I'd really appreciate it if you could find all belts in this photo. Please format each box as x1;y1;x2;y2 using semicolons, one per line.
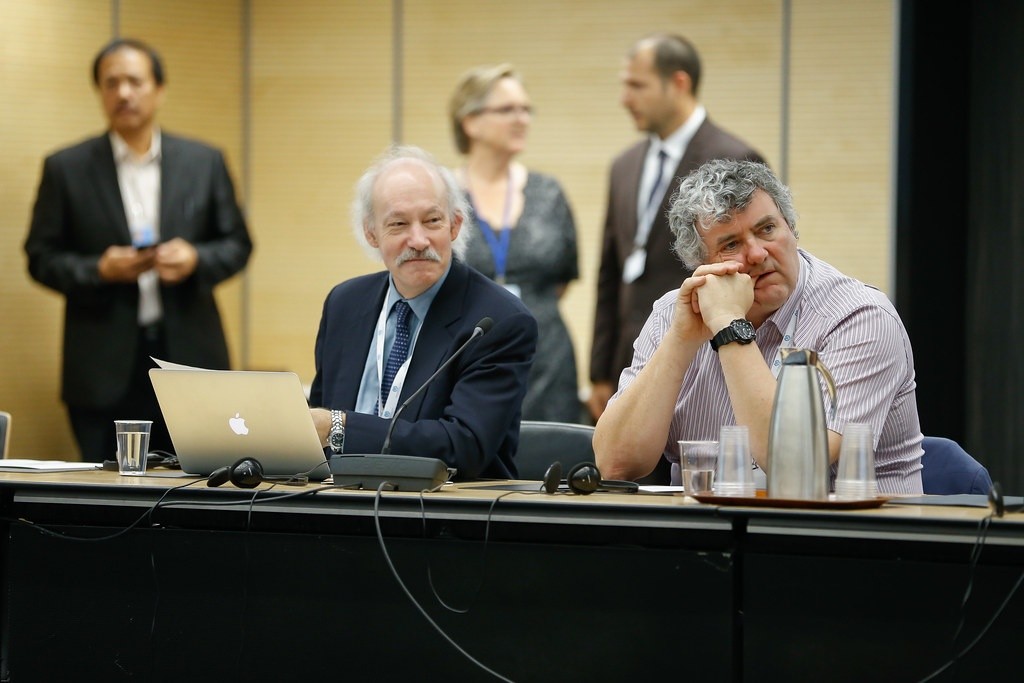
137;325;165;342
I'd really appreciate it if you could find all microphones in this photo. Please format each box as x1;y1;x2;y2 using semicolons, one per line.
330;318;494;491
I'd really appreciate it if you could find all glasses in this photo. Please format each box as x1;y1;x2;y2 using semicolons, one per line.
469;105;532;115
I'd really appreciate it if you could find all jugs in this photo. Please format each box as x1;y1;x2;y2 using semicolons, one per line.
766;348;836;502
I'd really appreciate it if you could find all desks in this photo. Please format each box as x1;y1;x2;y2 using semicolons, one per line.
0;462;1024;683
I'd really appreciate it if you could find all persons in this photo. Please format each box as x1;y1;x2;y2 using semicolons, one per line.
446;65;583;424
308;148;537;482
23;38;253;464
593;160;925;494
588;35;768;428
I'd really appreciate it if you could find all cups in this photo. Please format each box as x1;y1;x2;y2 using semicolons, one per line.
113;420;153;476
834;423;877;501
677;440;718;495
713;426;757;498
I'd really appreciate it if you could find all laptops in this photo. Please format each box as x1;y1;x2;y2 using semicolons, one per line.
148;368;332;482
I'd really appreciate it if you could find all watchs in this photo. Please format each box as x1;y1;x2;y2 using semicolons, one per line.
710;318;758;352
327;410;344;453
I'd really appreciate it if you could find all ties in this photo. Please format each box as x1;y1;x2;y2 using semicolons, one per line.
644;151;666;210
374;301;412;416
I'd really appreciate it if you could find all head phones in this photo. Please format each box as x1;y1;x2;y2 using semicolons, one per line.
206;457;309;489
103;451;183;471
544;462;639;494
986;482;1024;518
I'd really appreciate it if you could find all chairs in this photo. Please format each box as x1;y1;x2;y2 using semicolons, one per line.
919;434;994;496
510;417;668;478
